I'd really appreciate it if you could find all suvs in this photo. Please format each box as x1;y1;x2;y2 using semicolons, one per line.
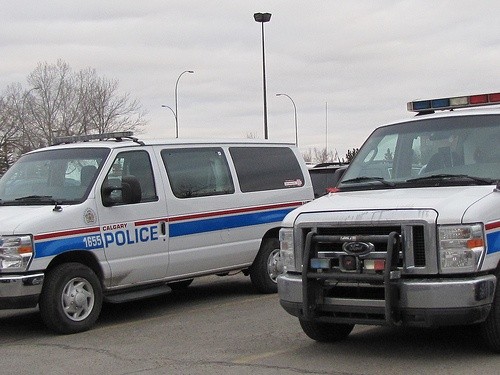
308;161;425;198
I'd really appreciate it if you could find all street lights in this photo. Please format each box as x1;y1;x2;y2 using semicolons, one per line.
253;12;272;138
276;93;298;148
162;70;195;138
21;85;40;154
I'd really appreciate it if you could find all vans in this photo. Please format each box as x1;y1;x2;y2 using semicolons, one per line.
277;93;500;354
0;130;315;334
305;162;319;169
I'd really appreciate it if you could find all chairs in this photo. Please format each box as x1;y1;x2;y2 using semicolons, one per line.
474;132;500;163
70;166;97;198
423;147;463;173
